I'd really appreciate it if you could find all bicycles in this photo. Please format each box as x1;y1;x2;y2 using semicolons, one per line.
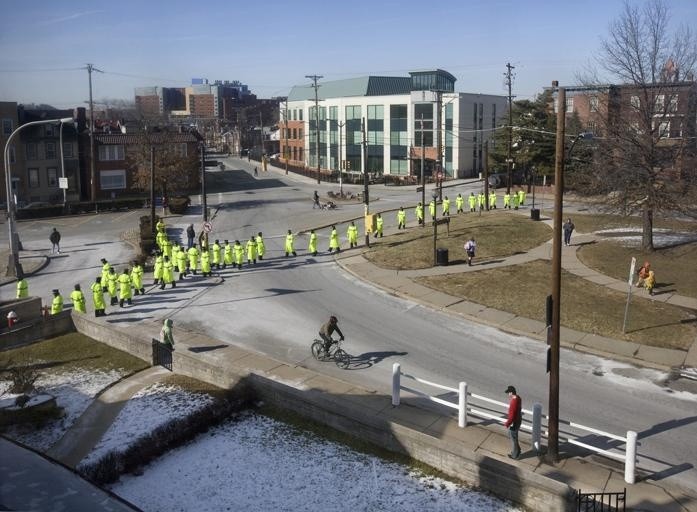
310;336;351;373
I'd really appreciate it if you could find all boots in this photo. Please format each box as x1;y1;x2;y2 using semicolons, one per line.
94;288;145;317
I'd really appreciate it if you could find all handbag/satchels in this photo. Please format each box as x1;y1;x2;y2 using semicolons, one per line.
467;246;475;257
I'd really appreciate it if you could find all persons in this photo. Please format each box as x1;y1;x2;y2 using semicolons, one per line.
643;270;655;295
254;167;258;176
159;318;174;346
51;289;64;314
50;228;61;254
504;386;522;459
563;218;575;247
15;274;29;298
319;316;344;357
635;261;651;288
70;284;86;314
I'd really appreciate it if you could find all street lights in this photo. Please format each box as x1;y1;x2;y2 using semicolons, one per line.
3;116;76;281
143;124;161;232
195;136;209;225
545;82;597;464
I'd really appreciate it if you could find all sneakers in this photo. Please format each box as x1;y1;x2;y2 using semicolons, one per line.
637;267;644;275
564;243;570;246
508;449;521;460
153;255;264;289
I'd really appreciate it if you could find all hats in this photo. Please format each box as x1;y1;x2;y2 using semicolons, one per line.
51;289;59;294
505;386;516;394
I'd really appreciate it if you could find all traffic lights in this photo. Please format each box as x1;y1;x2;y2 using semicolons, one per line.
432;171;436;181
347;160;350;170
438;172;441;182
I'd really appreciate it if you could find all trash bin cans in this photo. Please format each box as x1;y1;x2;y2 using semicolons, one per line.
531;209;539;220
437;248;448;266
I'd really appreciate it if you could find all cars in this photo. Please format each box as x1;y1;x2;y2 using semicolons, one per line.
270;153;279;160
